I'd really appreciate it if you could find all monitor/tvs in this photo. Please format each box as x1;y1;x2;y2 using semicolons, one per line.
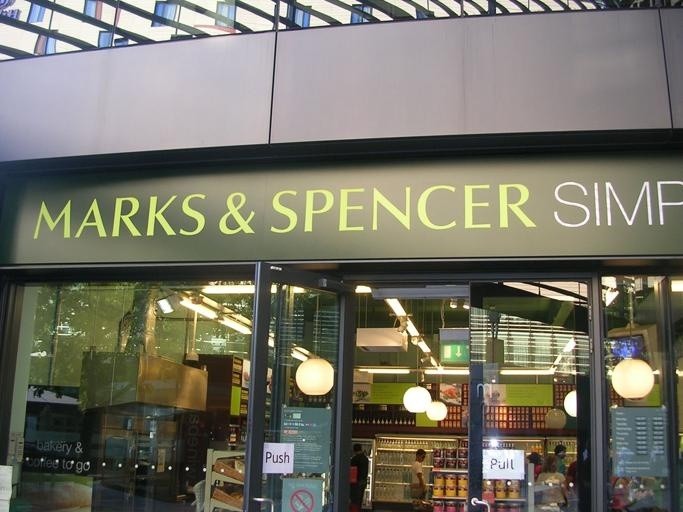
605;329;657;376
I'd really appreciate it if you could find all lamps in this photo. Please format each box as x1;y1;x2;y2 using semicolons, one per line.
176;289;313;363
396;312;431;364
424;374;447;422
384;297;431;354
610;276;655;401
563;388;577;418
294;291;334;397
402;299;432;415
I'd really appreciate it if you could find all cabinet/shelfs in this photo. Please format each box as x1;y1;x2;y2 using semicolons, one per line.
430;463;534;512
204;447;245;512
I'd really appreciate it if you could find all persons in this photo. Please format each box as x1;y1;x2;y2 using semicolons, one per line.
184;472;205;508
411;449;427;499
350;443;368;508
527;444;579;511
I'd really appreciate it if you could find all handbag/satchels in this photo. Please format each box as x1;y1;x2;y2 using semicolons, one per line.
350;466;358;484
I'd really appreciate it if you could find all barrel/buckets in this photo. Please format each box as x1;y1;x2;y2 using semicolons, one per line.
432;445;521;512
